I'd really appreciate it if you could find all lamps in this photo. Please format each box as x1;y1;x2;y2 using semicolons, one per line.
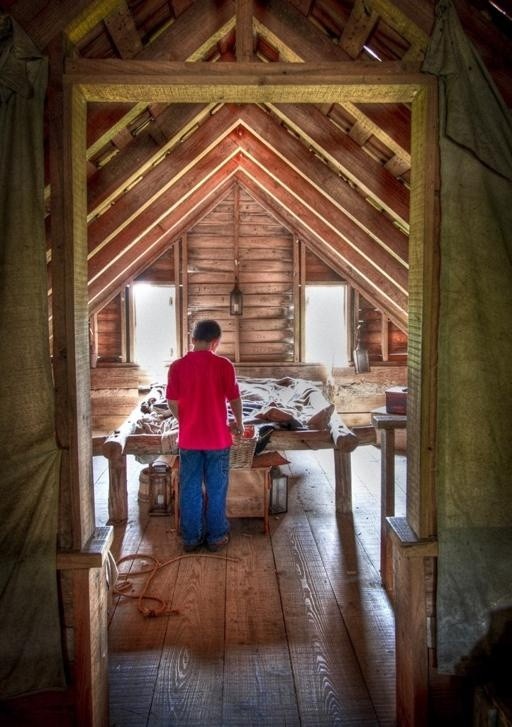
229;278;244;315
351;318;370;374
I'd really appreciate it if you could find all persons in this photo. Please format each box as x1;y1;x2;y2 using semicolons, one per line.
167;319;244;552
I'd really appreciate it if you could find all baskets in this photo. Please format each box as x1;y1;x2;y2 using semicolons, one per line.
230;425;259;469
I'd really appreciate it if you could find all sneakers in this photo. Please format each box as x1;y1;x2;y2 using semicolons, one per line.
185;534;230;552
